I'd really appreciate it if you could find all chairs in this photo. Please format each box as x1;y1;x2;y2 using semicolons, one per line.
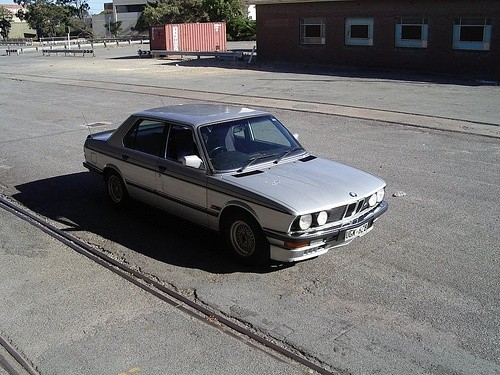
206;126;236;157
170;128;194;161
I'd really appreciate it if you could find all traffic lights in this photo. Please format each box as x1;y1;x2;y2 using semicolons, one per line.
65;26;70;33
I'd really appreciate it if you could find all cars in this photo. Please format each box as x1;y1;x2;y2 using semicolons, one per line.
82;104;389;265
